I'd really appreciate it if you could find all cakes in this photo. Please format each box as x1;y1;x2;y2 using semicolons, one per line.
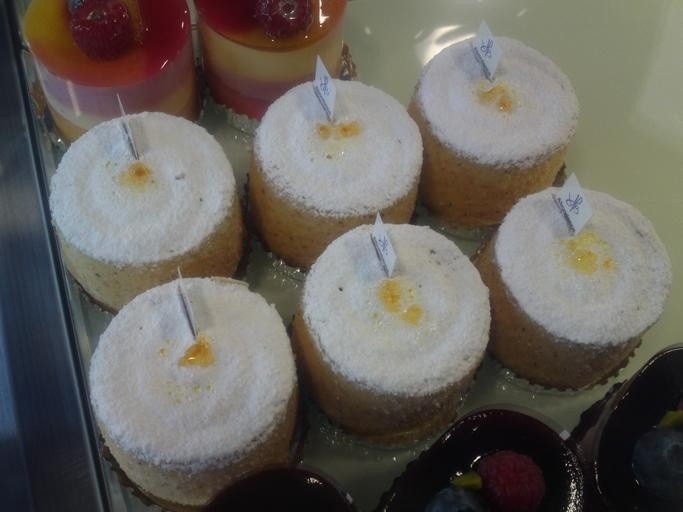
289;224;490;444
86;277;298;507
193;0;350;137
20;0;202;148
469;186;673;393
404;37;581;230
198;461;353;512
47;111;250;322
570;343;683;512
374;406;587;512
251;79;425;275
